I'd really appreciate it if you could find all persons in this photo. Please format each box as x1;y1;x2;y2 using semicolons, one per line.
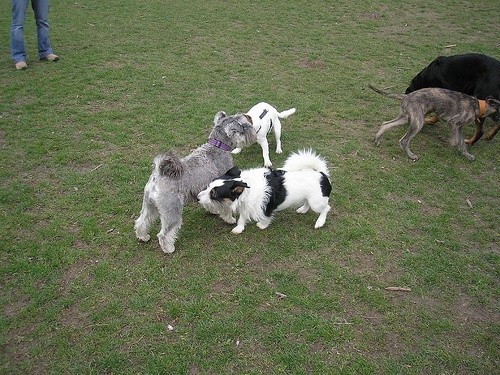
10;0;59;70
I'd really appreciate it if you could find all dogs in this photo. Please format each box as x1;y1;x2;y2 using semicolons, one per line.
366;84;500;162
196;146;332;235
133;111;258;254
231;101;297;168
405;53;500;147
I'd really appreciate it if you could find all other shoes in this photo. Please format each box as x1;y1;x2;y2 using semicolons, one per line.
15;61;27;70
46;53;60;62
